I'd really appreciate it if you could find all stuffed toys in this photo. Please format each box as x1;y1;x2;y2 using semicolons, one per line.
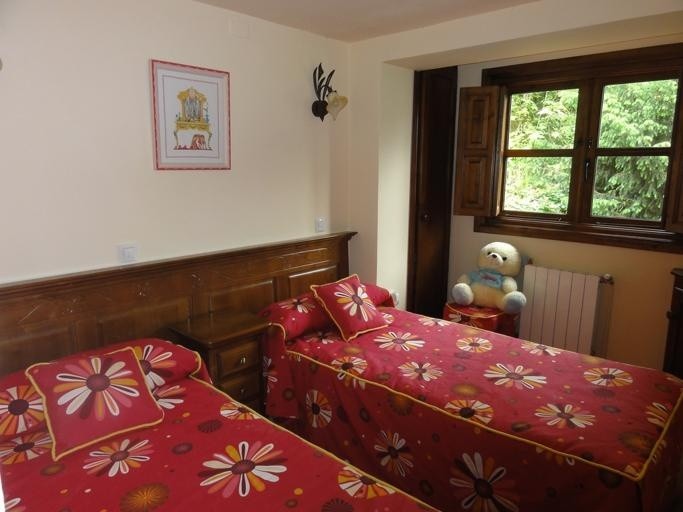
451;240;527;314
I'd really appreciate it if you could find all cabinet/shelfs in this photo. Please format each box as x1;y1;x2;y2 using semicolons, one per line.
161;308;273;411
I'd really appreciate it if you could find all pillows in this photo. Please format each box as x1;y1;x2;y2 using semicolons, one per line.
259;282;389;342
1;337;199;439
26;346;166;460
310;273;387;338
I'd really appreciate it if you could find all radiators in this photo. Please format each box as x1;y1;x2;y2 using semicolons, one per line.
518;256;615;356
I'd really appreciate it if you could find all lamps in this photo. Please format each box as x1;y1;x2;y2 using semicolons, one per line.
310;61;348;122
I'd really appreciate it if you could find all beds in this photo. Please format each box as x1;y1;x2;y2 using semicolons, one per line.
261;308;683;511
2;376;437;511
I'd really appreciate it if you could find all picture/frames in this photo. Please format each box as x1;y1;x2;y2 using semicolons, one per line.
150;58;231;171
